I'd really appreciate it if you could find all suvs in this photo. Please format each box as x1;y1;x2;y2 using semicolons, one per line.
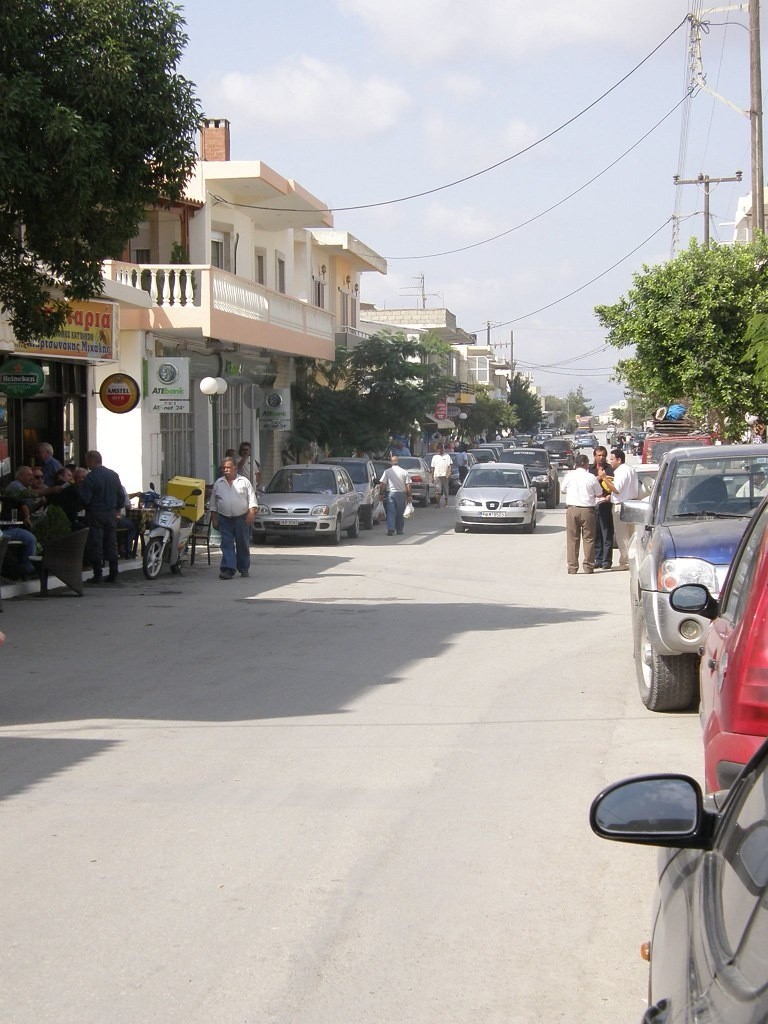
492;447;560;508
540;438;581;470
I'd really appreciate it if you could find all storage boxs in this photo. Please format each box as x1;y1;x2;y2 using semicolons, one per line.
167;475;206;521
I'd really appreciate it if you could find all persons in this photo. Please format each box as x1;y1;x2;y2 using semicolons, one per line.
0;441;136;586
236;442;262;491
431;436;468;452
645;429;656;438
617;432;634;454
754;424;768;462
735;464;768;498
560;445;639;574
209;457;258;580
215;449;236;477
395;442;411;457
454;448;468;483
430;443;453;508
354;448;369;460
379;456;413;536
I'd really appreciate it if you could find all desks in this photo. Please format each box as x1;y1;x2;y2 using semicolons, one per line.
127;508;157;559
0;521;23;568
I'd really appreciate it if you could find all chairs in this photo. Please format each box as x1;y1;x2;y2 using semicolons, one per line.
188;507;212;566
306;473;331;490
678;476;728;514
351;471;364;481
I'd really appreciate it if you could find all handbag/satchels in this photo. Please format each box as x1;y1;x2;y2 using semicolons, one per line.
403;501;414;519
373;501;387;521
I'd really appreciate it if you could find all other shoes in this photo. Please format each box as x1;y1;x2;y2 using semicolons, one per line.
110;576;122;582
387;530;393;536
241;571;249;578
396;531;405;535
612;565;630;571
86;575;104;584
602;564;609;569
220;569;234;579
593;564;601;569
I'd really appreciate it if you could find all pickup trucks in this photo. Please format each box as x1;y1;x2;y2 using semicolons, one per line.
620;440;768;713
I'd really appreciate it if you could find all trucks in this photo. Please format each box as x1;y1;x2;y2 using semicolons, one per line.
576;415;594;434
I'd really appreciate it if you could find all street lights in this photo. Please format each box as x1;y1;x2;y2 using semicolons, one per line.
458;412;468;441
200;376;228;546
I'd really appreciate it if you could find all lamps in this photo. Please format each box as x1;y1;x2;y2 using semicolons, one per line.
319;265;326;280
352;283;359;297
343;275;350;289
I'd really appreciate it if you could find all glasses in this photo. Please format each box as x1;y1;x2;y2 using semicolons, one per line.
33;475;46;480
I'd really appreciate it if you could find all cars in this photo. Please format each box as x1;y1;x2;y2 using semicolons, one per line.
251;463;363;545
395;456;436;507
589;732;767;1024
606;419;717;536
577;434;600;449
667;493;768;795
424;426;571;493
453;461;540;534
371;459;392;505
313;457;382;529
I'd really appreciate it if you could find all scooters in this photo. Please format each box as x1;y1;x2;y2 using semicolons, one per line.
136;482;202;580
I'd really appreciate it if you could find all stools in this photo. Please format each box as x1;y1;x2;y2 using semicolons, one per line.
29;556;48;597
115;528;130;560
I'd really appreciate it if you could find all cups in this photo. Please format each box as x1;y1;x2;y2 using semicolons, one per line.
11;509;18;523
